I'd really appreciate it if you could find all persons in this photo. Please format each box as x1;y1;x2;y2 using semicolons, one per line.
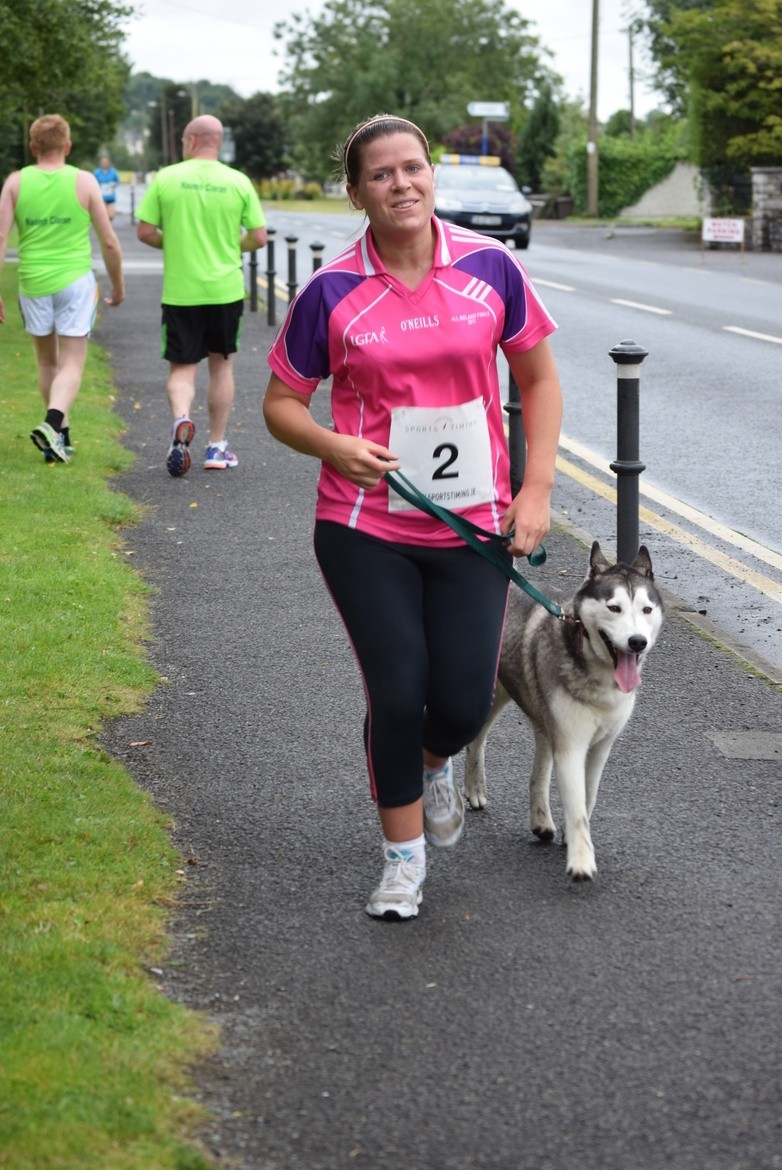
87;154;121;222
1;112;127;470
135;112;268;477
256;114;566;926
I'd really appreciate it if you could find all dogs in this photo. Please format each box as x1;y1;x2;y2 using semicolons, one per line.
460;537;663;882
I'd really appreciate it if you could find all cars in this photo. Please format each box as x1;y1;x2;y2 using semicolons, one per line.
431;154;534;249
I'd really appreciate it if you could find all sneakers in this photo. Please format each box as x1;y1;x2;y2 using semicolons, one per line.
422;755;465;849
364;853;427;922
166;413;196;478
204;444;239;469
30;420;75;465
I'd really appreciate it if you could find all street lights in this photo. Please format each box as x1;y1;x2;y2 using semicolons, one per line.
147;85;198;167
